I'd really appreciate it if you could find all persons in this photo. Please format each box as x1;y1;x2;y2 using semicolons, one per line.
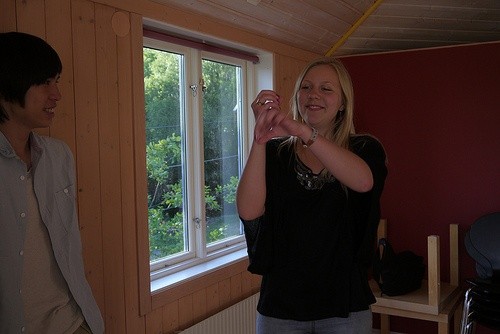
0;31;106;334
237;59;388;334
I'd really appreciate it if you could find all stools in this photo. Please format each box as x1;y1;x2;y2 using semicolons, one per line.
371;290;464;334
369;219;463;316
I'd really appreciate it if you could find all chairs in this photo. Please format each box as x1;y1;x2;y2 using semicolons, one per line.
460;212;500;334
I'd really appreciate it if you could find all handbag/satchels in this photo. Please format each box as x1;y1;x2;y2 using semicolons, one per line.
372;238;424;297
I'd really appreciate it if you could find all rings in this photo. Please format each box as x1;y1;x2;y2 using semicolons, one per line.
256;102;264;106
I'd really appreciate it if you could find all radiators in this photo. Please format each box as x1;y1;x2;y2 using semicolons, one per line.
177;292;261;334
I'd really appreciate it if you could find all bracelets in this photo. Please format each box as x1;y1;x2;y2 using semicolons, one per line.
301;127;319;151
